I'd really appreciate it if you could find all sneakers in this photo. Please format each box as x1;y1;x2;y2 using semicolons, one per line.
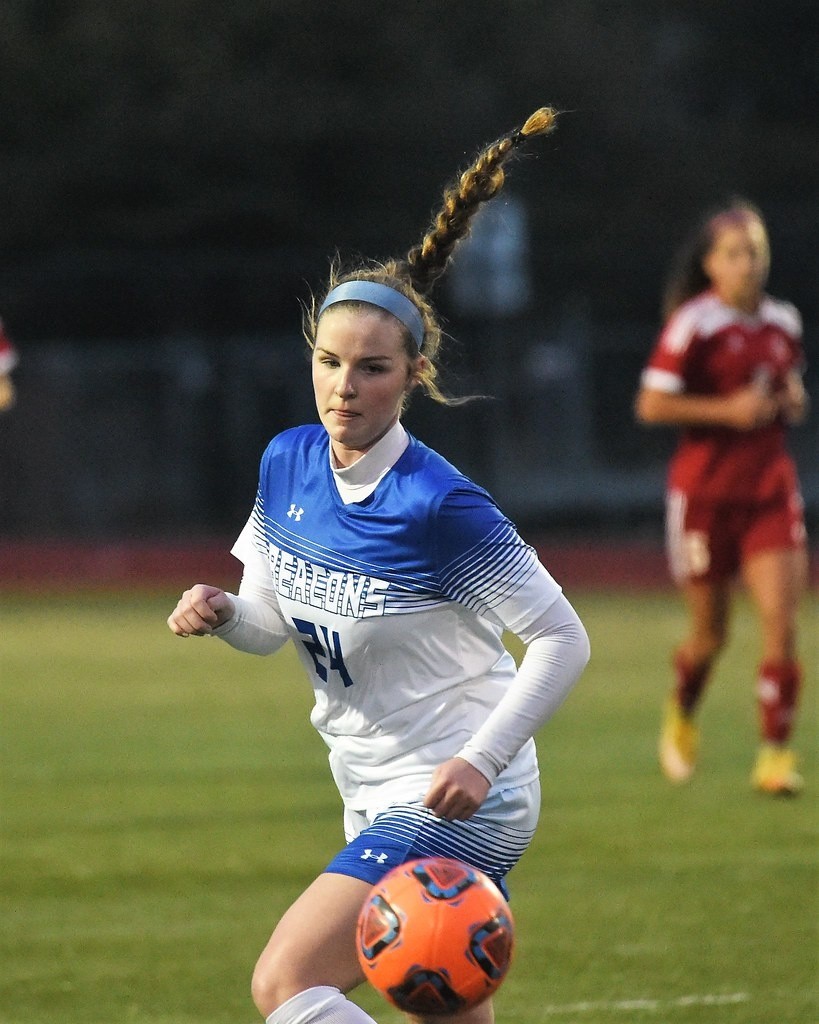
659;699;696;782
753;747;803;795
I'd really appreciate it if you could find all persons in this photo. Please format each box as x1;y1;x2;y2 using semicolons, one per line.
635;200;811;798
166;106;590;1024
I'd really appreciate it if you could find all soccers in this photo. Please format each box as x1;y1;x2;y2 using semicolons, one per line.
357;858;518;1016
